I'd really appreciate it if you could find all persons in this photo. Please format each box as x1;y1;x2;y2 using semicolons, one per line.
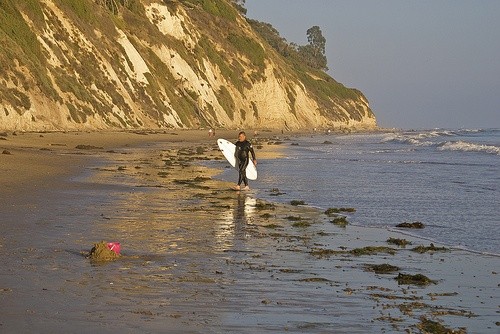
208;128;216;137
234;132;258;191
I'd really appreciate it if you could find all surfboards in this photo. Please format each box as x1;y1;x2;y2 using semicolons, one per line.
217;138;257;181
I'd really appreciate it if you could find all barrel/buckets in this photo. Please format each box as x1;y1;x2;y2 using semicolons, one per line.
107;242;120;255
